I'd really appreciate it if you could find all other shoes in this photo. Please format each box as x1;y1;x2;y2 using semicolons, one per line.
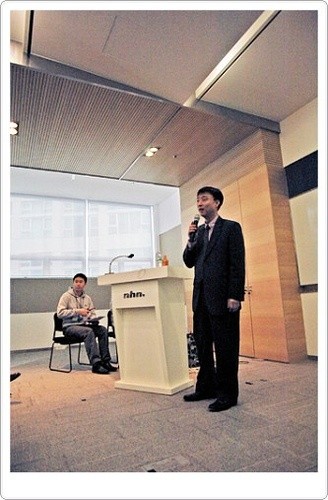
102;362;119;370
92;365;110;374
208;400;235;412
10;372;22;381
183;393;213;402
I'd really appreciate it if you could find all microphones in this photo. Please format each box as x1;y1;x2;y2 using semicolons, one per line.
189;215;199;242
109;254;134;273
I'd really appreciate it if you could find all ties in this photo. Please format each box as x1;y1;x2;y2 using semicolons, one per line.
202;224;211;255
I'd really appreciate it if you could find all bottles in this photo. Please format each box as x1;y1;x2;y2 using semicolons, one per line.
156;251;162;267
162;255;169;266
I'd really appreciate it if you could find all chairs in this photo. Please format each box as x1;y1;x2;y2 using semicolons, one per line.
49;309;118;373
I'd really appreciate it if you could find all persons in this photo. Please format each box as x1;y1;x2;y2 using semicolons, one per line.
57;273;117;374
183;186;245;412
